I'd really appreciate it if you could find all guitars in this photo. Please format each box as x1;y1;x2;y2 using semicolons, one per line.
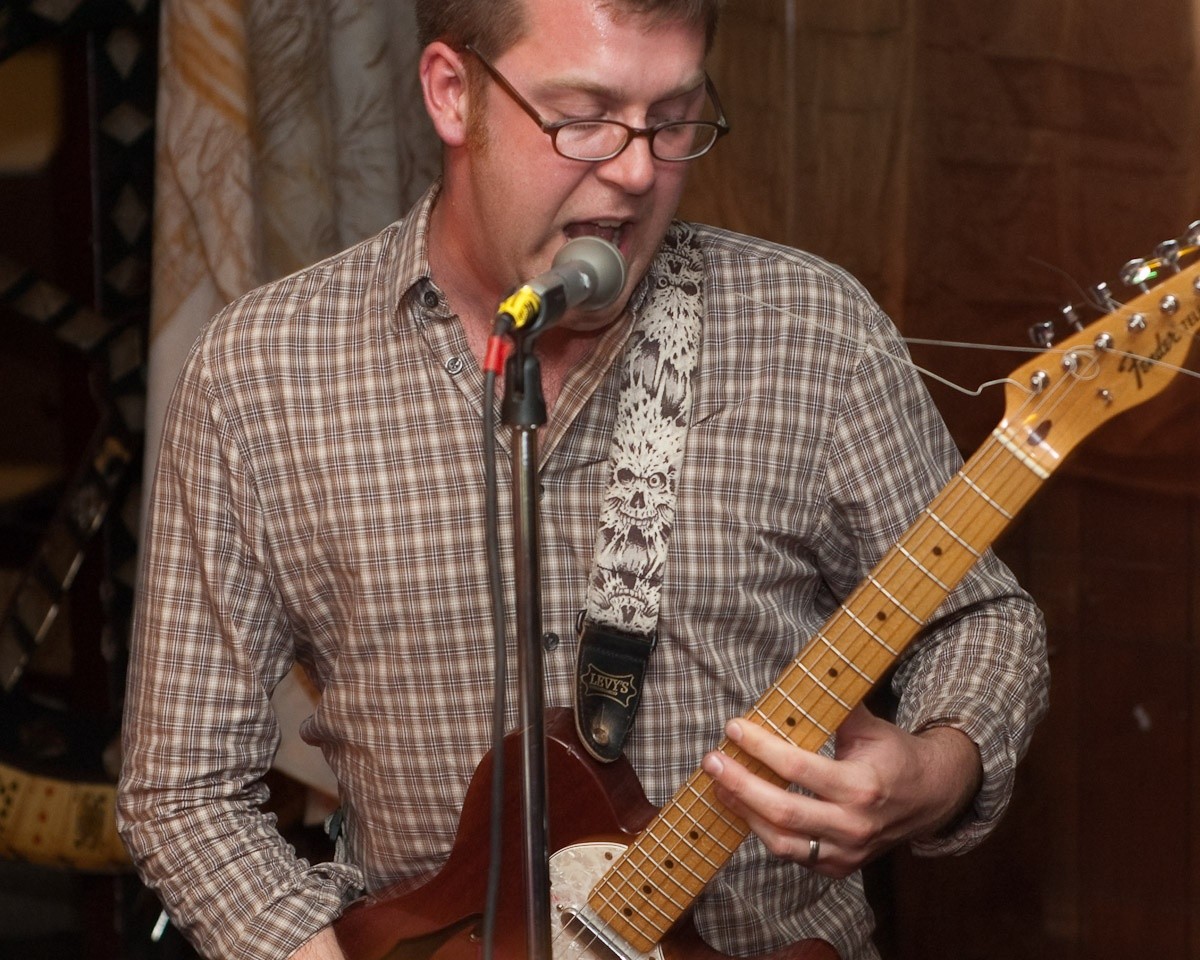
331;219;1200;960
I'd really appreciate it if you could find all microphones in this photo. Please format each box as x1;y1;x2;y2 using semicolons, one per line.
498;236;628;332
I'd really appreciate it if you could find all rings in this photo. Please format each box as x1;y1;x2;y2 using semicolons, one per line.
805;838;819;867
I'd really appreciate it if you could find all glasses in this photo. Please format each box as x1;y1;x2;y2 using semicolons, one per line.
448;42;729;164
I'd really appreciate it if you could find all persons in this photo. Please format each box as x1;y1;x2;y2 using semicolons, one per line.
111;0;1048;960
